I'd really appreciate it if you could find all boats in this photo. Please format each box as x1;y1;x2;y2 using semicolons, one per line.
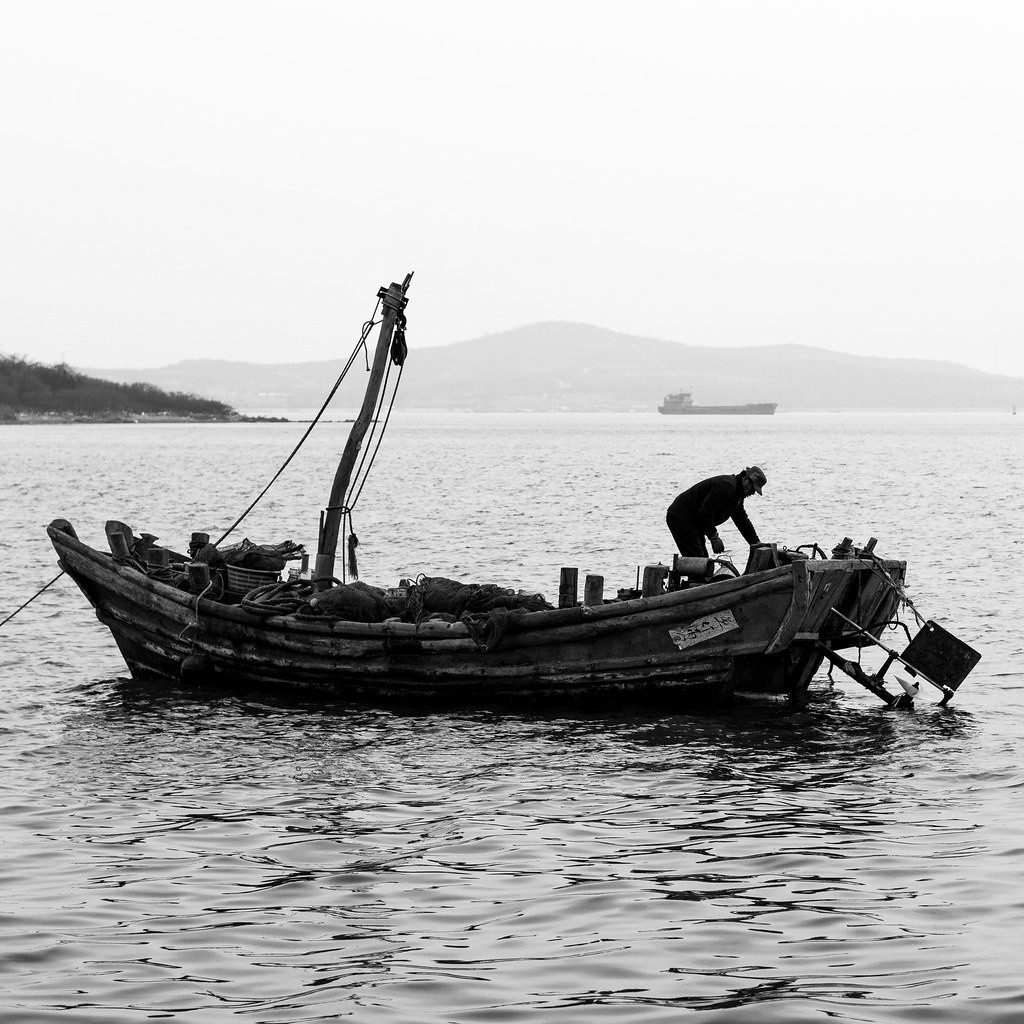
657;391;780;416
44;271;984;721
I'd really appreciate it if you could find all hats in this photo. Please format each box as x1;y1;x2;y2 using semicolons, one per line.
744;466;767;496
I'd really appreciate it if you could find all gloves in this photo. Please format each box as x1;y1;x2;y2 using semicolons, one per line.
710;532;725;554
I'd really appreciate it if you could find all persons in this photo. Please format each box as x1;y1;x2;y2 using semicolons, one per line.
666;466;768;581
